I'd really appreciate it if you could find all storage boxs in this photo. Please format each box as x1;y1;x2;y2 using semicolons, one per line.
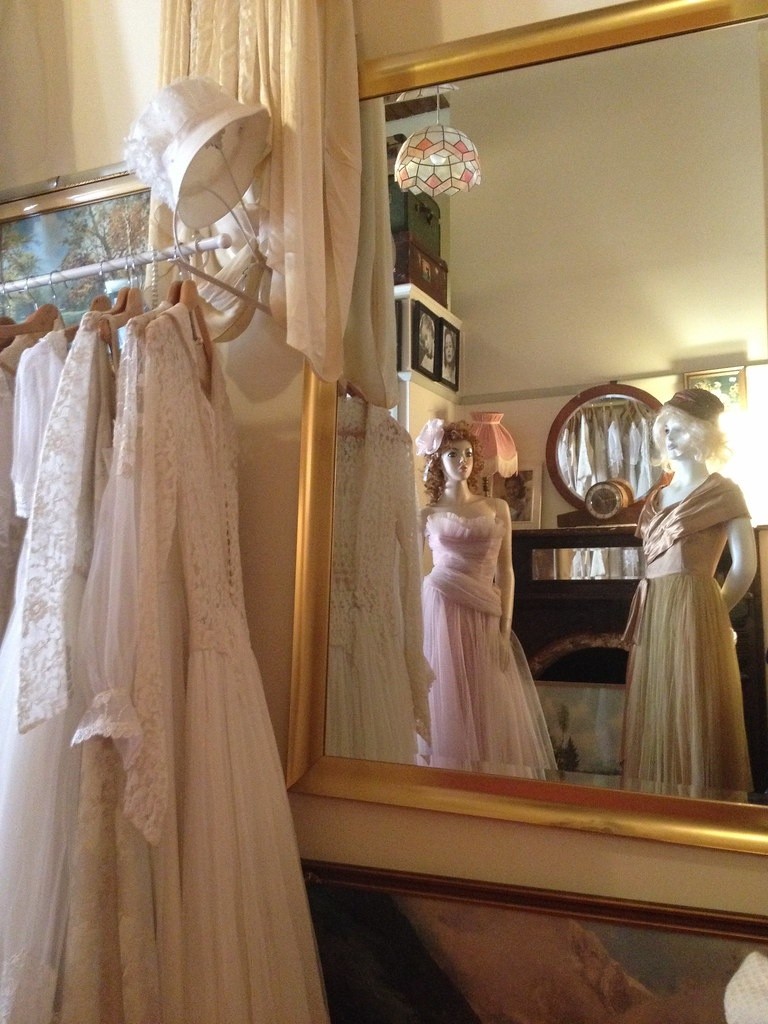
387;229;450;311
388;173;443;261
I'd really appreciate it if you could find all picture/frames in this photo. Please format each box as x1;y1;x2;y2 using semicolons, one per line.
490;466;545;531
0;165;166;348
684;364;748;410
438;316;462;388
412;298;442;378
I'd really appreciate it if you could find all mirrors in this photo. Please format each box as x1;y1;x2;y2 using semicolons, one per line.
289;0;764;841
548;378;676;530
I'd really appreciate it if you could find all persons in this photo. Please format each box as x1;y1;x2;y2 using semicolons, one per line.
418;417;556;771
622;388;756;794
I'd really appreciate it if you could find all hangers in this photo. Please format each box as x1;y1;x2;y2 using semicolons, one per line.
560;394;658;444
337;369;416;447
2;242;236;413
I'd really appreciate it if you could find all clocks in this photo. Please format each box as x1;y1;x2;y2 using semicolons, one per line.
555;481;642;526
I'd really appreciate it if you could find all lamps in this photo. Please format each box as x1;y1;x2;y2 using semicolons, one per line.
390;80;481;204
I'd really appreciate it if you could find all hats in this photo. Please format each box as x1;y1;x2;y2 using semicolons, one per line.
668;389;724;419
126;77;270;229
